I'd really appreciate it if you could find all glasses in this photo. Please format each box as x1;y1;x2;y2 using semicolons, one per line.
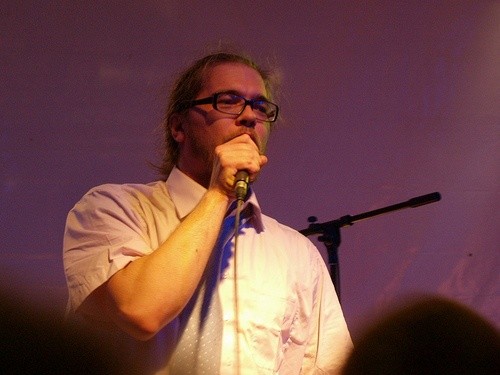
178;93;279;122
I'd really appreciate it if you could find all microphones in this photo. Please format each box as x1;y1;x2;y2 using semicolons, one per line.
235;170;250;200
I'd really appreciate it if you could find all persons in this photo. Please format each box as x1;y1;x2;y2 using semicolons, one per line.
334;294;500;375
63;50;356;375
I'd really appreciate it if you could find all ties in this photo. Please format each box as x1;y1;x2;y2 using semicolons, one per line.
163;215;253;375
